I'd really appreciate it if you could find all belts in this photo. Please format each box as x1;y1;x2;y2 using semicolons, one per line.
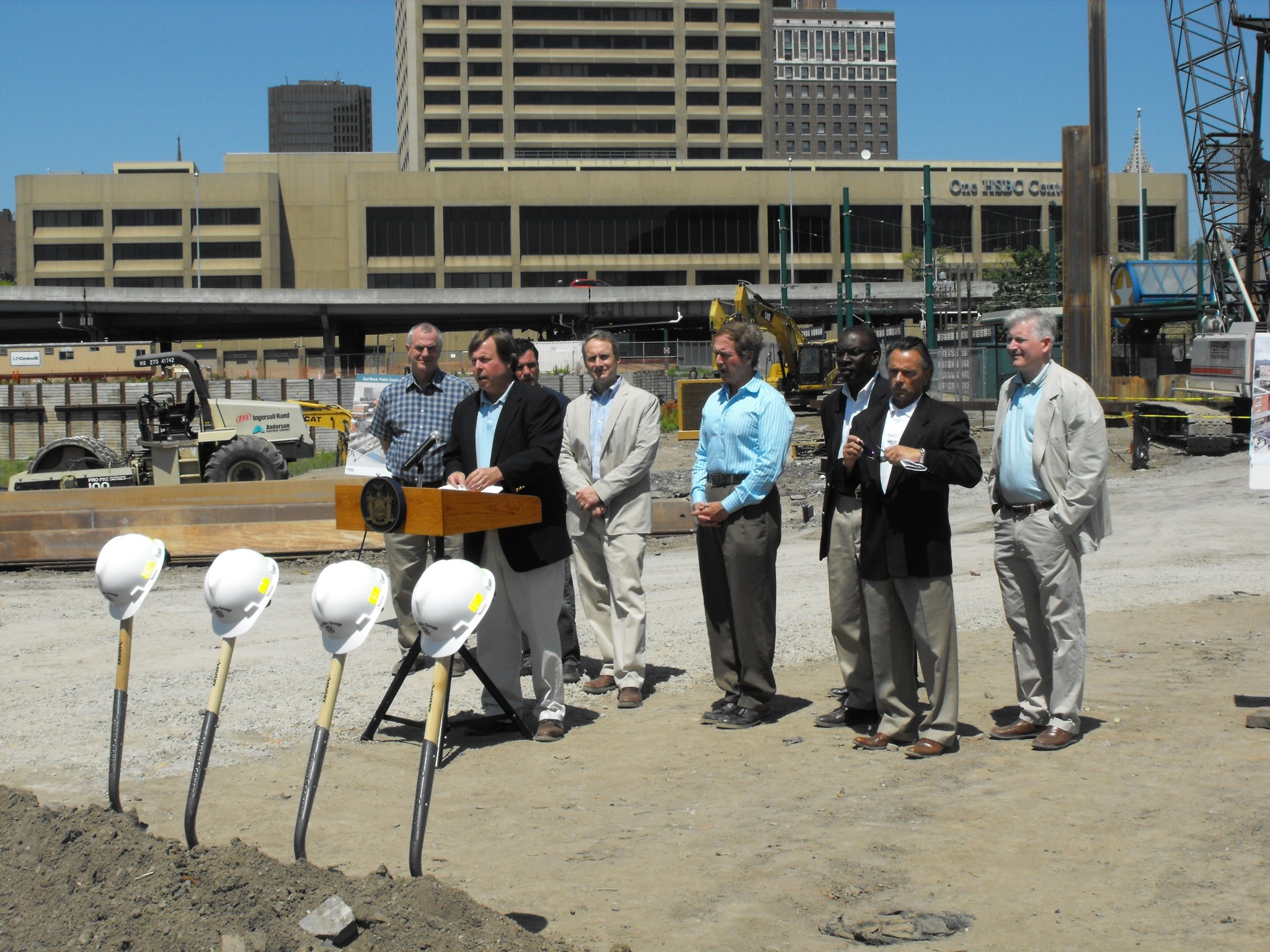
1001;499;1053;513
392;476;447;489
705;473;745;487
838;489;862;500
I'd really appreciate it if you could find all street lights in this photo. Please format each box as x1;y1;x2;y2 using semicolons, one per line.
788;158;794;284
194;169;201;289
1136;108;1143;260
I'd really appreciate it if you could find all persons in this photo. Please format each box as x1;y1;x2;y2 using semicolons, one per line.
514;338;581;684
813;324;919;728
688;322;795;728
367;322;476;676
989;307;1114;750
828;336;983;758
442;327;573;741
557;329;661;708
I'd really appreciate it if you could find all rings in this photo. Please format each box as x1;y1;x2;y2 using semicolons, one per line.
891;455;896;459
479;481;483;485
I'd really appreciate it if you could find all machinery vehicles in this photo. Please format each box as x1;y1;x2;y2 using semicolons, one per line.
6;353;354;491
1131;1;1270;470
676;282;842;439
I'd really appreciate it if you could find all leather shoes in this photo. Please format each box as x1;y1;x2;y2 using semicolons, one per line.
904;738;947;758
464;716;520;736
1031;725;1080;750
699;701;739;724
852;732;913;750
535;719;564;742
827;687;850;697
813;706;870;727
989;718;1047;739
617;687;643;708
583;675;617;693
716;702;773;729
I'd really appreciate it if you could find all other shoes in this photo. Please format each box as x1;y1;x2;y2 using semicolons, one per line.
392;656;427;675
452;658;467;676
520;655;533;676
562;665;581;683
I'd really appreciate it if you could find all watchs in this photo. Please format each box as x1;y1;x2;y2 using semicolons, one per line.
919;448;925;464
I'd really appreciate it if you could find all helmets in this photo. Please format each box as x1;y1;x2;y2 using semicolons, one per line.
95;533;165;621
204;548;280;638
411;559;496;657
310;560;390;653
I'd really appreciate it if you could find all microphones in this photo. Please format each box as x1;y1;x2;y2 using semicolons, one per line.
402;430;443;472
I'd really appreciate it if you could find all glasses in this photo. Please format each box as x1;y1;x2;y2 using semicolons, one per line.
857;420;888;462
830;348;874;359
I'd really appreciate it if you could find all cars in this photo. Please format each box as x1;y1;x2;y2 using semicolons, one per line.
569;278;609;287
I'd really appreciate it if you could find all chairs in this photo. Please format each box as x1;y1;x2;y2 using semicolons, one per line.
159;388;195;429
